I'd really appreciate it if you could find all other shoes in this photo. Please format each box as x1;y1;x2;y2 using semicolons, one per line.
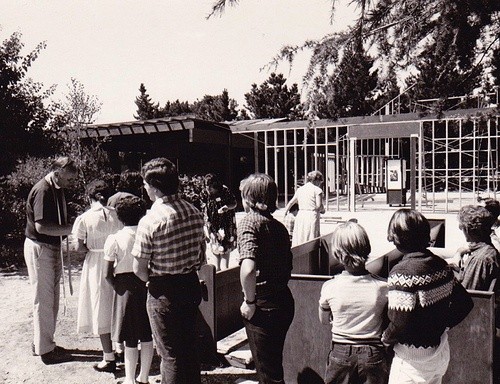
94;359;116;373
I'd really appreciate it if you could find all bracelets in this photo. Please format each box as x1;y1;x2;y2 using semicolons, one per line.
243;296;258;304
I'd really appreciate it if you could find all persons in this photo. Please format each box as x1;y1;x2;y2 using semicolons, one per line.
236;173;295;384
284;203;300;248
23;157;80;364
390;170;398;181
199;173;237;272
317;221;389;384
131;158;207;384
283;171;326;249
106;170;144;202
71;179;125;372
104;195;154;384
479;198;500;252
447;205;500;384
380;208;474;384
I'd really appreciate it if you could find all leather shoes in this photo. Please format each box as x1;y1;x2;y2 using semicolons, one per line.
41;347;74;365
